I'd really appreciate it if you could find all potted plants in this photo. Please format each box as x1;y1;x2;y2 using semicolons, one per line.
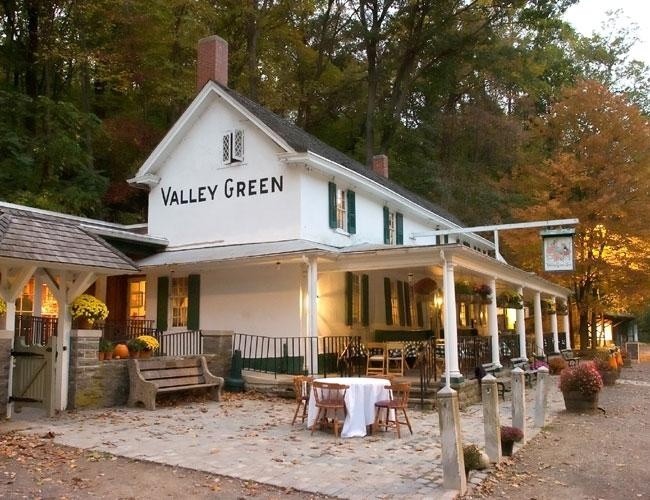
128;335;160;359
500;426;524;456
548;357;568;375
68;294;110;329
463;443;482;482
557;361;604;413
530;360;550;379
590;347;631;385
99;337;113;361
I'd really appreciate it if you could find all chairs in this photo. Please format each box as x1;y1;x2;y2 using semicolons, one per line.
291;338;445;444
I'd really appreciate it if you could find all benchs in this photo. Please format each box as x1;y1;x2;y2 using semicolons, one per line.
560;349;580;370
481;363;511;401
511;357;538;389
127;356;224;411
